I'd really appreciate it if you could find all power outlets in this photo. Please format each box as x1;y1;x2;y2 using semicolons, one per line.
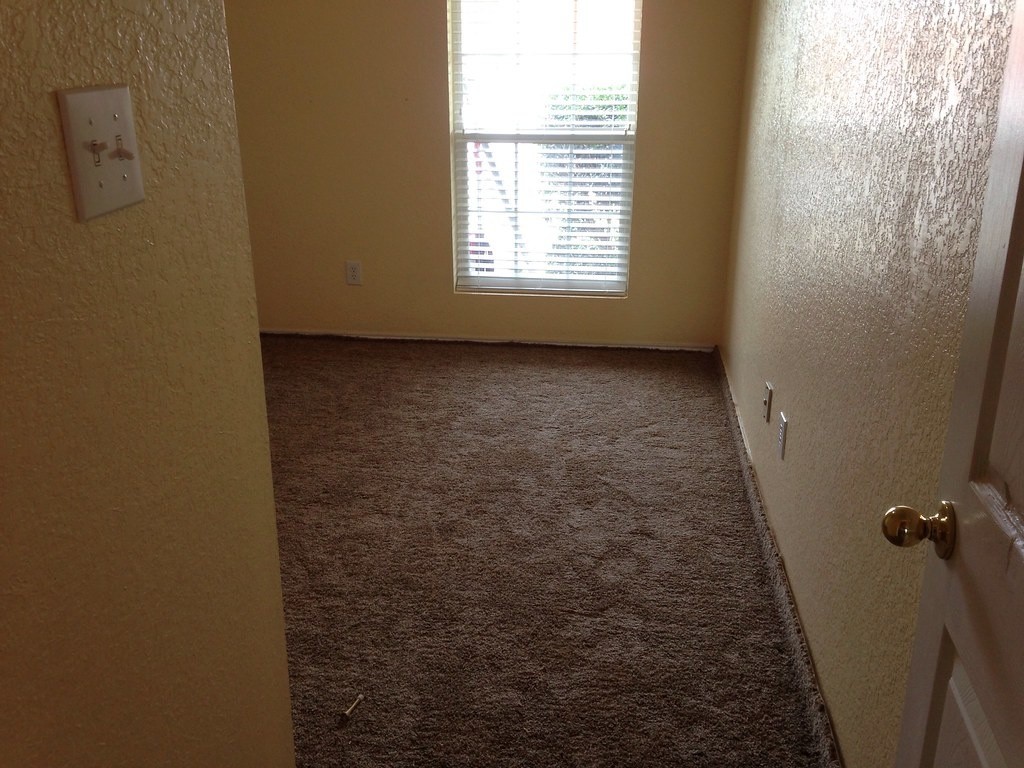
347;260;362;285
777;412;787;459
